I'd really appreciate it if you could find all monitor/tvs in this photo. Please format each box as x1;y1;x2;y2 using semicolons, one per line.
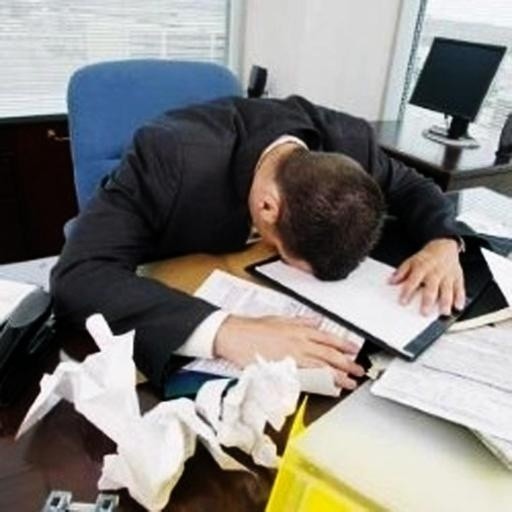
408;36;507;147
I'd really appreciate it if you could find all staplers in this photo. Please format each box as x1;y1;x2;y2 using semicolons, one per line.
0;289;54;389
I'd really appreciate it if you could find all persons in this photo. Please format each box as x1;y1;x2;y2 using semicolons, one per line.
49;95;467;390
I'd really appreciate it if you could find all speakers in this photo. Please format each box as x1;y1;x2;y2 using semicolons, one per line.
247;64;267;97
495;112;512;157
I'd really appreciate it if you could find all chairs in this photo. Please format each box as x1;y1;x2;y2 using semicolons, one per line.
66;58;244;214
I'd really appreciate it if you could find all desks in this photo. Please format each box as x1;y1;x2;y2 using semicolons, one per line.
369;118;512;200
0;237;347;512
